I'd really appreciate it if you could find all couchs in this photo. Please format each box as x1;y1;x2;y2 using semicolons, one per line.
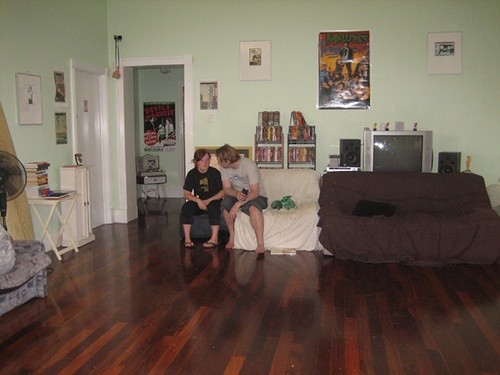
317;171;500;266
0;224;52;317
486;183;500;217
230;169;324;251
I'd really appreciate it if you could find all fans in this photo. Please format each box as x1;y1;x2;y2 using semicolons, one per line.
0;150;28;233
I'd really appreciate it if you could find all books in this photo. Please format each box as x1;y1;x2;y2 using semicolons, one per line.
256;145;284;166
287;145;314;167
44;190;77;199
254;111;285;144
287;110;316;143
23;161;50;200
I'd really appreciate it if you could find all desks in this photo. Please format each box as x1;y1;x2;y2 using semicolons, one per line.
28;192;80;263
136;170;167;203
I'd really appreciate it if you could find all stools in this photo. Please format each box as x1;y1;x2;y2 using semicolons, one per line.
179;210;230;242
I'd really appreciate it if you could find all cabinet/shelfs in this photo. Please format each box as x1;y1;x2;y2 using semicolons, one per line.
286;132;317;171
254;133;284;169
59;166;96;247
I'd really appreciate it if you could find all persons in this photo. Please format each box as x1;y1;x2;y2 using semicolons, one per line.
215;143;268;254
180;149;224;248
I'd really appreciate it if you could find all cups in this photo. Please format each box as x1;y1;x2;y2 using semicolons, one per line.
241;188;248;201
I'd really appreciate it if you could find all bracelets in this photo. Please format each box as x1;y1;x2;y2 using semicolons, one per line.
235;191;239;199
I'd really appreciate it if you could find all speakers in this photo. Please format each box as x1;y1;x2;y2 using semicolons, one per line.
339;138;361;167
438;151;461;173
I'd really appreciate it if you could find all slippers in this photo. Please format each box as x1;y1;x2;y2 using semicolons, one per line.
202;241;219;248
184;240;195;248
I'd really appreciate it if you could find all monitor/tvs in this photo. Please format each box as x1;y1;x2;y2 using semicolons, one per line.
363;131;432;172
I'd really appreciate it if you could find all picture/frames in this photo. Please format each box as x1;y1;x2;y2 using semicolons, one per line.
15;73;44;126
239;40;272;82
52;111;69;147
427;31;463;76
194;146;253;161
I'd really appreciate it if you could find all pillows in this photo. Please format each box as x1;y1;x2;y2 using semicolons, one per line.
351;200;397;218
442;190;483;216
